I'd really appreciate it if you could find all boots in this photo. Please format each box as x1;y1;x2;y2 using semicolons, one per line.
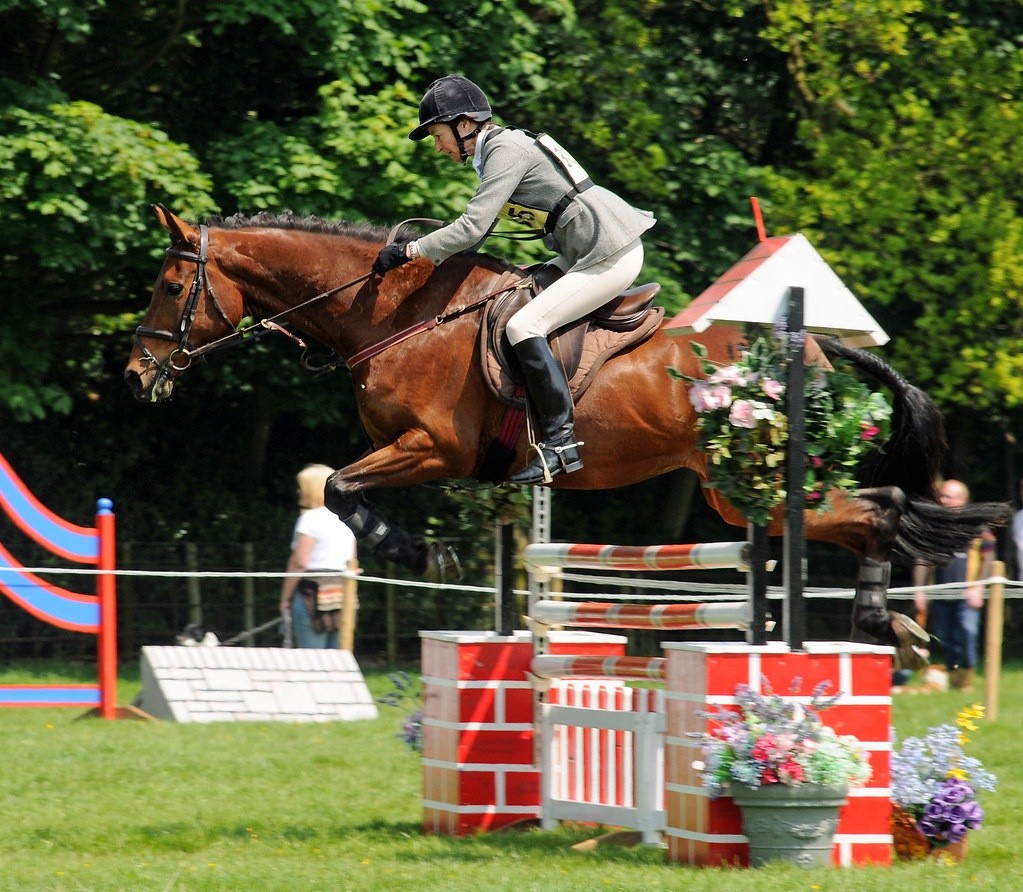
505;336;585;483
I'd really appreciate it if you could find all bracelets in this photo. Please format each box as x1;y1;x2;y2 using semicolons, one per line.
409;241;415;259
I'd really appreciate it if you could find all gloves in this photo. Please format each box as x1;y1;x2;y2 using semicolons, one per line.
372;241;413;277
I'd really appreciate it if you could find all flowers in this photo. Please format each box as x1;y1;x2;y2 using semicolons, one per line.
687;671;872;791
879;710;999;841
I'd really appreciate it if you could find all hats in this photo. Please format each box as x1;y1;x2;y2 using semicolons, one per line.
408;74;492;140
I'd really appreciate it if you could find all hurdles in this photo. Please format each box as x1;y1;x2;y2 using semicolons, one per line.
520;476;771;854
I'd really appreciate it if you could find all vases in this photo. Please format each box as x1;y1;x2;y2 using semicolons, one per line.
730;784;847;865
890;825;969;864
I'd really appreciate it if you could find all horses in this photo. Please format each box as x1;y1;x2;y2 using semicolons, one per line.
121;202;1015;650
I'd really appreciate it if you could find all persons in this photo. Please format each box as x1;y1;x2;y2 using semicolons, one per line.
913;480;1023;694
370;78;657;486
279;464;358;648
